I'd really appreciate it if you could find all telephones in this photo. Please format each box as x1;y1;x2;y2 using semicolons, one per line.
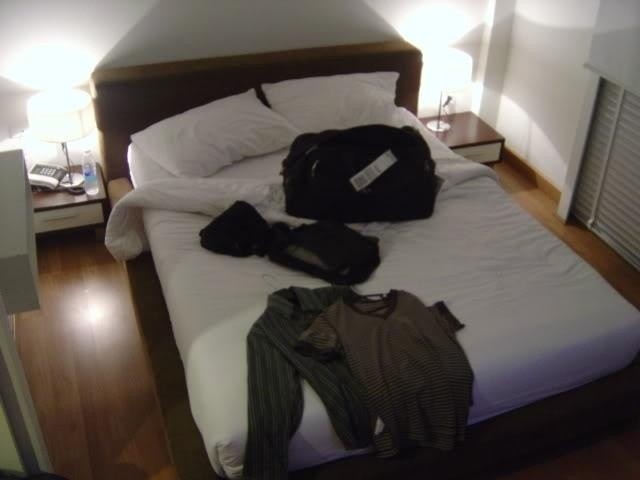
27;162;68;191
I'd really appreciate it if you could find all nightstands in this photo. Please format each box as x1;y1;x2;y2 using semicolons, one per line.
26;162;106;237
418;112;504;169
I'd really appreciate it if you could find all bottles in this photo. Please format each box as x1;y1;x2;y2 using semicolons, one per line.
83;148;99;195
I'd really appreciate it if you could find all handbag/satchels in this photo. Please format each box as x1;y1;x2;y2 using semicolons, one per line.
270;216;382;286
281;124;442;222
198;201;274;259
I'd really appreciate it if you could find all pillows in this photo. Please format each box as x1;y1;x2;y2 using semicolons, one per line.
128;70;401;181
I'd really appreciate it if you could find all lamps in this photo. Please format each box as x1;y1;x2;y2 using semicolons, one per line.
40;104;86;188
422;48;474;132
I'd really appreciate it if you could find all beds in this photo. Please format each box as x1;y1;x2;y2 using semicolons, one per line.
90;41;639;480
